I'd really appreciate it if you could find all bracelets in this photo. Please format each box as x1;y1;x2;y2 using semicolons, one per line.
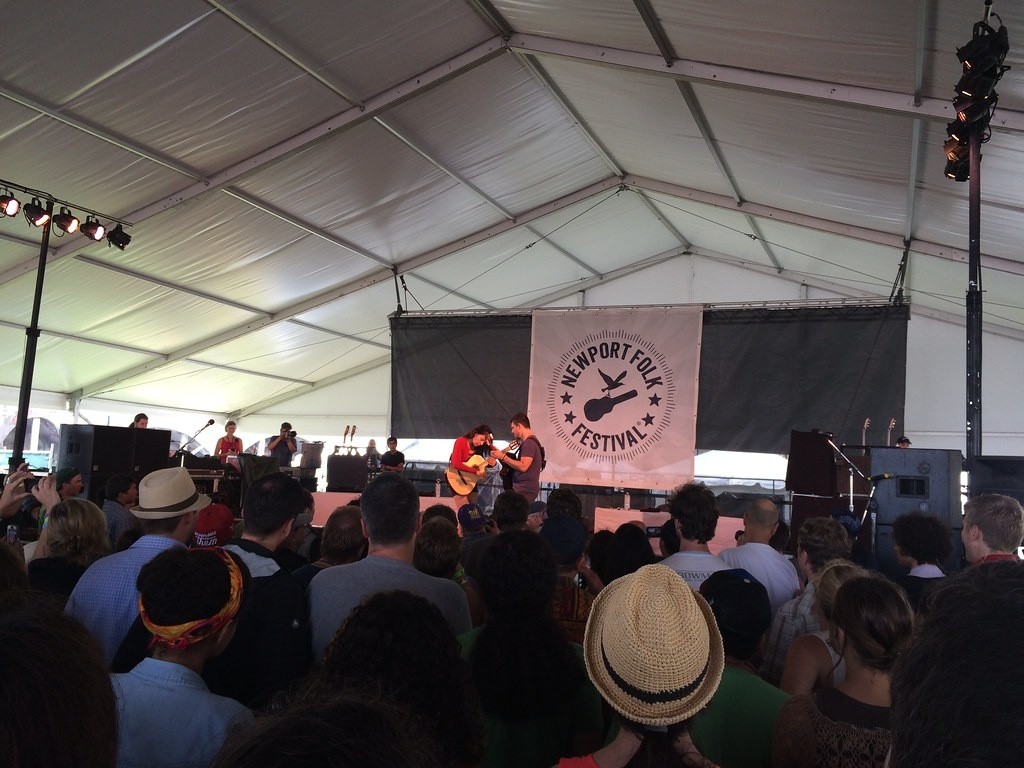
501;455;506;462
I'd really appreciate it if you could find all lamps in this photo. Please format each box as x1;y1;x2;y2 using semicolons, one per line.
956;62;1007;100
957;88;999;124
52;206;80;234
943;133;984;162
23;196;51;227
956;21;1009;78
0;187;21;217
80;213;106;242
946;112;990;145
945;153;982;182
106;222;131;250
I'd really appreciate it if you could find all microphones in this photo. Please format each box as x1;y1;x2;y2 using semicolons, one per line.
344;424;356;445
202;419;214;431
867;471;897;481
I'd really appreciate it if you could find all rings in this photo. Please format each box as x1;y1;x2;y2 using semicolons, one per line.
6;478;13;484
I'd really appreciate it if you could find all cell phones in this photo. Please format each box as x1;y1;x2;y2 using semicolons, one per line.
647;527;662;537
24;478;40;492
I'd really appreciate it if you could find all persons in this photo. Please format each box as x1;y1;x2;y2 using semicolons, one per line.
0;413;1024;768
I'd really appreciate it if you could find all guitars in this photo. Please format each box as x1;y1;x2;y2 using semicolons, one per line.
886;416;896;446
862;416;870;455
442;439;522;497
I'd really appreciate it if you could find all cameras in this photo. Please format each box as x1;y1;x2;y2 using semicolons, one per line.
289;431;296;437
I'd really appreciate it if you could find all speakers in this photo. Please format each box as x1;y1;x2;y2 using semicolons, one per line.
868;448;963;529
56;425;170;510
971;456;1024;509
875;523;959;579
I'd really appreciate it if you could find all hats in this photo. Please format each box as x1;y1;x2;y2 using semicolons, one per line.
56;467;81;483
583;564;726;726
191;504;236;547
130;466;214;519
700;568;772;637
458;504;485;528
529;501;546;515
